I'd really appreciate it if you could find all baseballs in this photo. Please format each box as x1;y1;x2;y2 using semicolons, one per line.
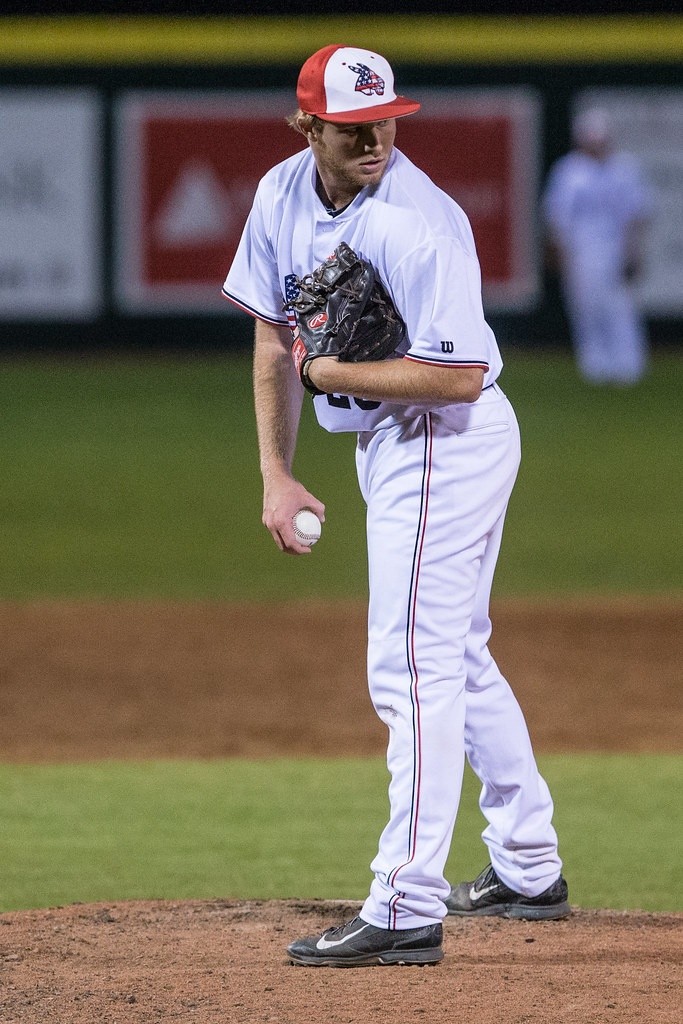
292;509;323;547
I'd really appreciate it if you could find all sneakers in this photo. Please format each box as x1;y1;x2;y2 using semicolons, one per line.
287;915;444;968
444;862;572;920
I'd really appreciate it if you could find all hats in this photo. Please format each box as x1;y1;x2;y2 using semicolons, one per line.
296;45;421;123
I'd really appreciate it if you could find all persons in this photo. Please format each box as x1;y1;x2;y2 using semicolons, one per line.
541;108;651;384
220;43;568;967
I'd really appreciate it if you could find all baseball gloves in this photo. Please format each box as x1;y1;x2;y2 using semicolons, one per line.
280;239;408;400
